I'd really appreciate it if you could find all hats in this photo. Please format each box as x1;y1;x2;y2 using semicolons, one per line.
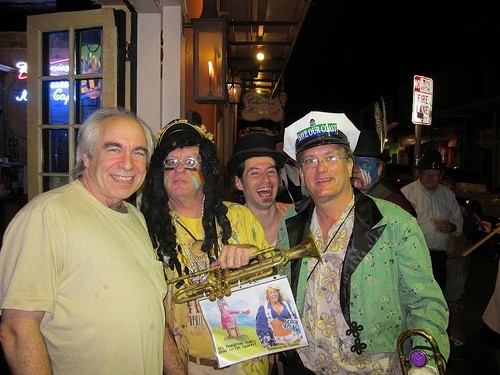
414;151;446;169
227;133;287;174
353;96;398;162
154;119;214;148
284;111;360;161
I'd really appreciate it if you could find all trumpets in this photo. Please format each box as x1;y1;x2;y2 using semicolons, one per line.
166;230;324;305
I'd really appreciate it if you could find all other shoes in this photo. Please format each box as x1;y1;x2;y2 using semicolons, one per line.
450;329;466;347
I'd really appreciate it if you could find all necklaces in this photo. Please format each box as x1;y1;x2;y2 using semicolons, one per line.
167;193;206;283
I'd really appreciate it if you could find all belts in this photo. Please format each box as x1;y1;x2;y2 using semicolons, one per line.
189;355;217;367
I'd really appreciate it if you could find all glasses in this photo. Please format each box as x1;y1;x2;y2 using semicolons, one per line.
162;157;200;169
302;155;348;168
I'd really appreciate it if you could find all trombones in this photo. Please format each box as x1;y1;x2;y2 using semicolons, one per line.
397;329;445;375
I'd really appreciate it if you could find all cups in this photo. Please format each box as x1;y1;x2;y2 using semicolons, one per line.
435;217;447;230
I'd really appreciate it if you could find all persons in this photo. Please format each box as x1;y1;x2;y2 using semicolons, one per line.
255;286;302;350
0;109;167;375
401;158;463;293
275;109;450;375
225;133;294;248
350;128;417;218
140;119;276;375
0;109;500;375
